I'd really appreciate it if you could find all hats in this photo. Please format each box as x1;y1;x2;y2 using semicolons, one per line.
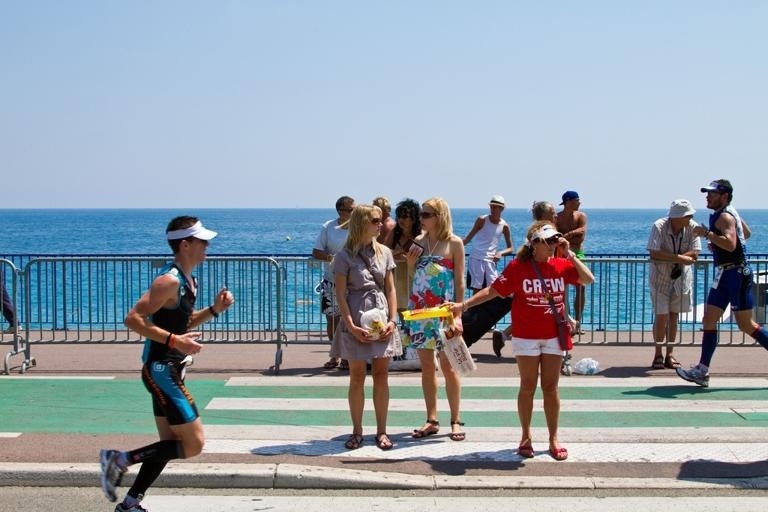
531;224;563;241
558;191;578;206
165;221;217;240
700;181;733;195
667;200;696;218
488;196;505;208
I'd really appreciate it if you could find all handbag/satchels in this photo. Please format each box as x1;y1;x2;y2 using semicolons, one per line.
555;315;573;350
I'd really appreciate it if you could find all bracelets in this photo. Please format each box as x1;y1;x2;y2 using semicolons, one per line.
165;331;176;349
461;300;468;313
208;304;219;320
566;251;576;261
704;229;710;237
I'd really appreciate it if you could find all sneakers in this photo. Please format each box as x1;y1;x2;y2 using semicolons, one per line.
493;328;505;357
677;364;710;387
115;503;147;512
100;448;127;501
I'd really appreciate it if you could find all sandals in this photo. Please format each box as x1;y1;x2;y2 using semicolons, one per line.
346;433;364;449
450;420;466;441
652;354;665;369
325;357;340;369
666;355;682;369
413;420;440;439
375;431;393;449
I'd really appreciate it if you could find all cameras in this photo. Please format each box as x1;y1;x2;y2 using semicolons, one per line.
670;266;682;280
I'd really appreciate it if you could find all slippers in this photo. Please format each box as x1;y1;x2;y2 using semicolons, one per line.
549;445;568;460
520;438;534;457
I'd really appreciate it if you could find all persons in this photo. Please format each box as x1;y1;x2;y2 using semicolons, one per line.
311;196;467;449
437;219;598;461
98;215;238;511
0;285;23;334
646;198;701;369
532;200;557;225
675;180;768;387
461;194;514;296
554;190;589;336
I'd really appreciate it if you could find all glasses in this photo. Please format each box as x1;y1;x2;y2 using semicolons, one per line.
542;236;559;244
396;214;410;219
339;208;353;212
419;212;436;219
368;218;383;225
